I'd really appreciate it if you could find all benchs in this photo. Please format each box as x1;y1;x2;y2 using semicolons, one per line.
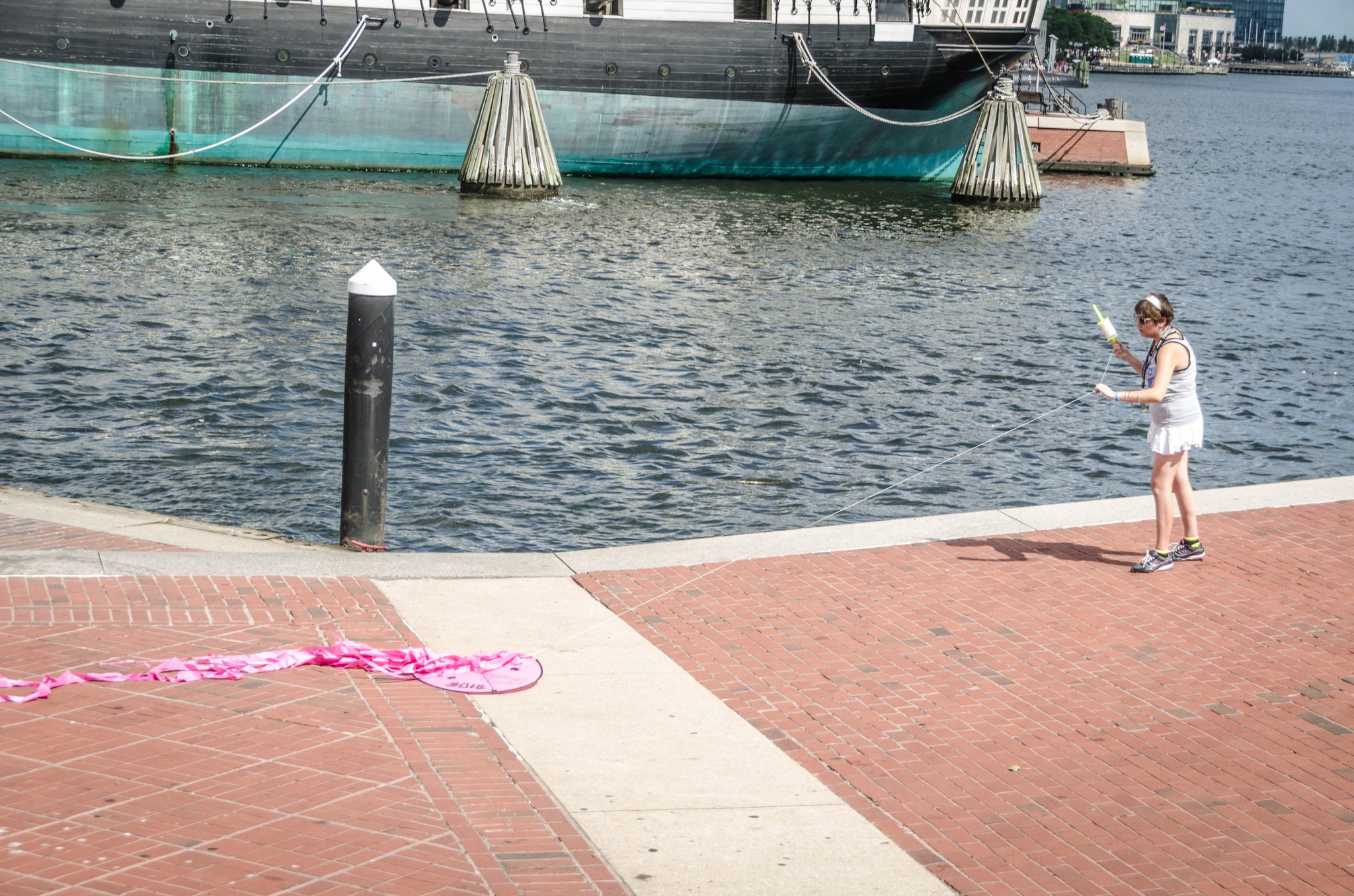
1014;90;1055;114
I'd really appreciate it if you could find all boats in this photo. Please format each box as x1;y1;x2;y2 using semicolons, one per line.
0;0;1048;183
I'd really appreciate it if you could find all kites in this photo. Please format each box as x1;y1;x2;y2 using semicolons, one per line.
0;640;543;705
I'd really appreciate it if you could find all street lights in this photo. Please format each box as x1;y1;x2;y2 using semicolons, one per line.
1074;42;1087;60
1068;41;1072;71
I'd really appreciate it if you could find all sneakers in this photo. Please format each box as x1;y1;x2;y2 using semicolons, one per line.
1170;539;1205;561
1131;550;1173;573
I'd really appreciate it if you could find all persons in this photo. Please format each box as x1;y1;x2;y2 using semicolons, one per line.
1095;293;1204;572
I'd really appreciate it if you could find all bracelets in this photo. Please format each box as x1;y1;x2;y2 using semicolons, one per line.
1115;391;1119;402
1125;391;1128;404
1138;389;1140;404
1129;390;1132;405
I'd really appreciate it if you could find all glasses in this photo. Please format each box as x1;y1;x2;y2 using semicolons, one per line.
1133;313;1153;325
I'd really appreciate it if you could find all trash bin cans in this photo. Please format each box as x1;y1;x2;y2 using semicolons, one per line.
1146;54;1150;63
1142;54;1146;63
1149;54;1154;64
1139;54;1142;63
1135;53;1139;63
1129;53;1135;63
